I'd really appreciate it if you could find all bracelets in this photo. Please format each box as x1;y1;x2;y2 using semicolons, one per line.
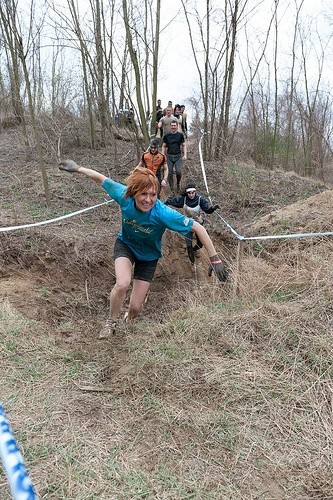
210;254;219;262
163;179;167;183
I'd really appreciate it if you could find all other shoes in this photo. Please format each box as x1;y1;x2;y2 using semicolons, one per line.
123;312;136;324
189;252;194;263
177;185;180;193
99;318;117;339
170;186;175;193
193;250;201;258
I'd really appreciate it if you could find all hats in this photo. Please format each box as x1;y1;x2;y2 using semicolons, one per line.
150;140;159;148
181;105;185;108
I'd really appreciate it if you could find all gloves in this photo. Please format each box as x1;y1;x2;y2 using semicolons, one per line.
161;179;167;186
207;253;229;282
207;204;219;214
58;159;80;174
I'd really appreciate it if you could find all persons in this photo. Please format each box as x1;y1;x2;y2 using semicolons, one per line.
58;159;228;340
163;101;174;115
152;106;178;138
162;121;187;192
173;104;188;139
130;139;169;200
156;100;163;138
164;184;221;274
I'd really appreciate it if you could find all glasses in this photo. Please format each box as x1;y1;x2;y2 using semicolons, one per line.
188;191;194;194
172;125;175;126
169;104;172;105
